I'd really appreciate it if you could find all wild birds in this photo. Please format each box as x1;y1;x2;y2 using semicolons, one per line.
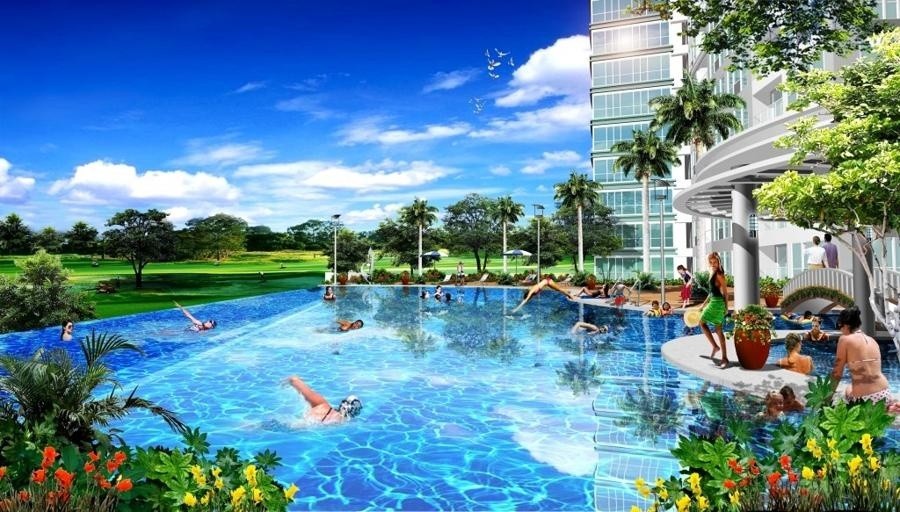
468;96;488;115
484;47;515;80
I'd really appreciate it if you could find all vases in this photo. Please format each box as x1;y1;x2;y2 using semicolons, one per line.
734;329;771;369
766;293;778;307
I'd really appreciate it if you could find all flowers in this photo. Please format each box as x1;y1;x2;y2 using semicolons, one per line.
764;285;779;298
726;304;778;346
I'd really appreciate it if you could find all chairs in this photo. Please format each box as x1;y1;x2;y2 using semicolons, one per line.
443;275;452;284
521;274;536;285
480;273;489;286
557;277;571;287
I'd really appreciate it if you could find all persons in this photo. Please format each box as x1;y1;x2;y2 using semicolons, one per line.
260;376;363;433
335;320;364;332
420;233;900;422
323;286;336;300
172;302;217;333
61;320;73;341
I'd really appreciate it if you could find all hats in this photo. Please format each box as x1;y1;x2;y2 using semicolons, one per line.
340;397;361;418
684;307;700;328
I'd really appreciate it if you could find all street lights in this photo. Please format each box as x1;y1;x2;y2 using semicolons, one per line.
534;203;545;284
650;179;671;310
330;214;340;287
